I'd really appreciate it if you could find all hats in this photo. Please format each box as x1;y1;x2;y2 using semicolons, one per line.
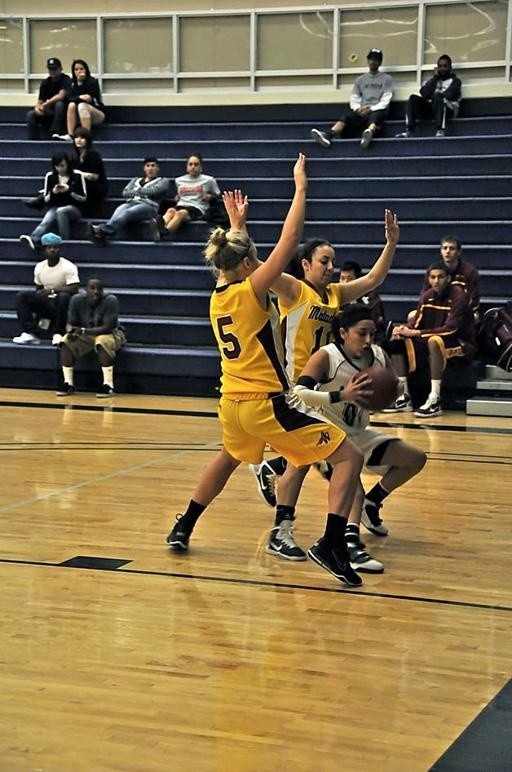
47;57;63;70
42;232;61;247
366;48;383;63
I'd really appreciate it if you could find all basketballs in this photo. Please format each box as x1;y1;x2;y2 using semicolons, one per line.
353;366;400;409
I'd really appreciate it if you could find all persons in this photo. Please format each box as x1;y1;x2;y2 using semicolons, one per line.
311;47;395;148
27;57;71;140
381;262;478;418
52;59;106;142
20;151;87;253
396;54;462;137
148;153;222;243
294;304;426;574
12;232;82;346
22;128;108;218
86;155;166;248
165;152;362;583
339;261;384;322
56;277;128;399
233;189;400;561
440;235;481;320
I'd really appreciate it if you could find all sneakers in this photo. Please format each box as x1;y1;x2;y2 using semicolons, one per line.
396;131;416;138
20;233;37;253
382;394;413;413
435;130;447;138
360;496;389;536
165;513;190;551
13;332;41;347
414;398;443;417
348;544;384;575
55;383;77;395
149;215;171;242
87;222;109;246
96;383;117;398
264;526;307;561
52;133;73;141
51;334;64;347
307;537;363;587
309;128;333;149
249;462;280;509
358;128;373;149
